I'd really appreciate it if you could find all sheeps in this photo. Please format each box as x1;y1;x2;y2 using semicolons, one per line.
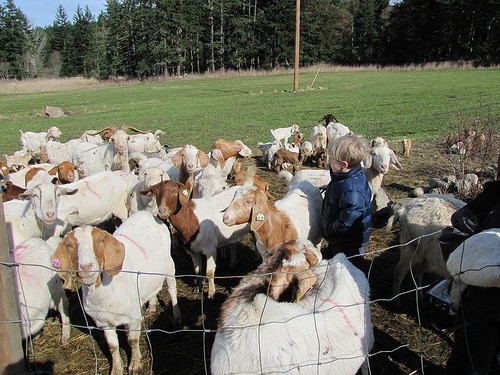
0;114;500;375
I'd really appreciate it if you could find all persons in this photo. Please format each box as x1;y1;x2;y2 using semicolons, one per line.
319;136;372;267
